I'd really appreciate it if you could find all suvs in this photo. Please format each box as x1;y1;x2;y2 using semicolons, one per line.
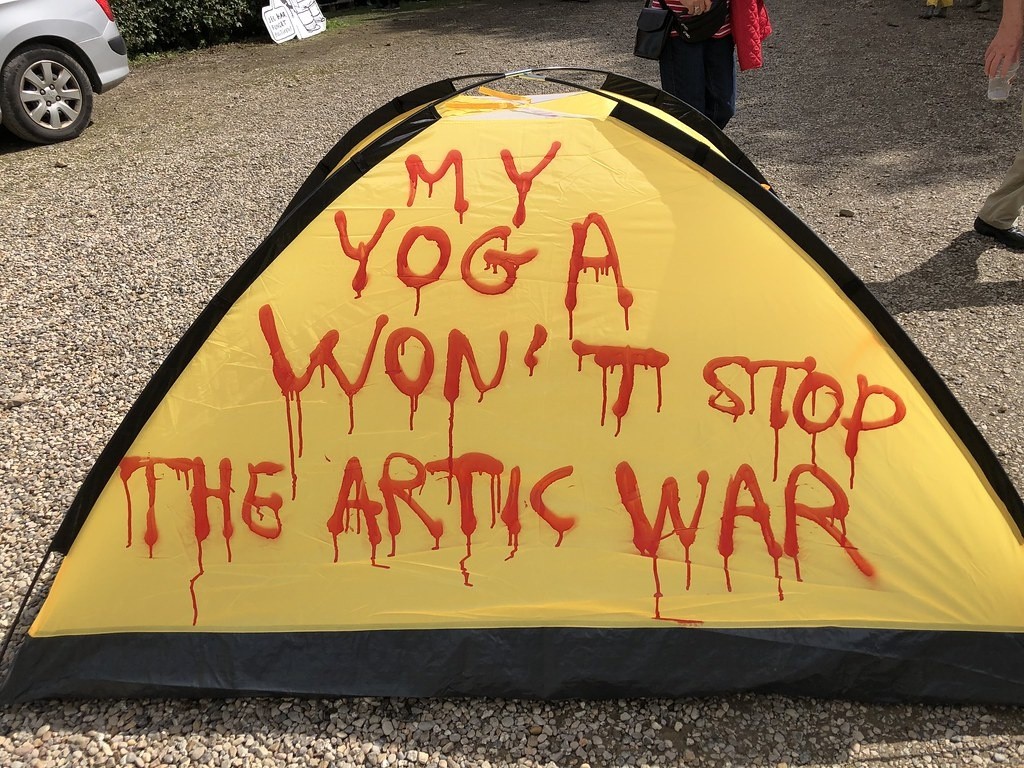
0;0;134;144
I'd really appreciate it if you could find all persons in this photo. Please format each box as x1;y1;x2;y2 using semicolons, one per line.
652;0;734;130
974;0;1024;250
922;0;953;18
967;0;990;12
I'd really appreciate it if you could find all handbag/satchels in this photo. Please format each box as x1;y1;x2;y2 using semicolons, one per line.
675;2;729;45
633;7;674;60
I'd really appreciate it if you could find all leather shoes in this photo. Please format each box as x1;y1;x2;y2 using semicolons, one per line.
973;216;1024;249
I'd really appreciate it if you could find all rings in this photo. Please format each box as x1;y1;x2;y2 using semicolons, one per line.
694;6;699;12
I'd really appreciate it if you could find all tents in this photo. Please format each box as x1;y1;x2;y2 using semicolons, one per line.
0;67;1022;709
